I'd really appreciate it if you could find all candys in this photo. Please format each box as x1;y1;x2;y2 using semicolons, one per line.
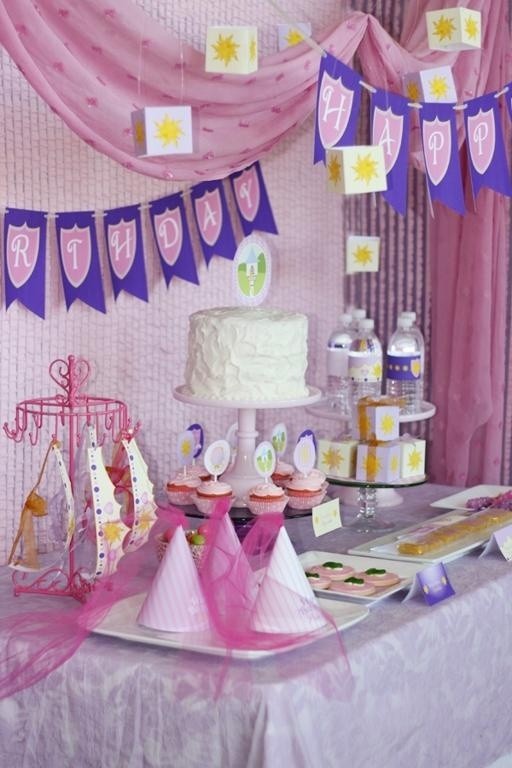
164;525;210;545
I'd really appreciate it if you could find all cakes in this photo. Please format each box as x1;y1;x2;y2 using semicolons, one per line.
166;462;328;515
184;307;310;402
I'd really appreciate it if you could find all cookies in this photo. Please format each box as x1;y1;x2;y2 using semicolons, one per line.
305;561;400;596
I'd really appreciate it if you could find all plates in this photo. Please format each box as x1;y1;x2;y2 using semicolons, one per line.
79;573;369;662
430;484;512;509
349;509;512;564
252;550;431;602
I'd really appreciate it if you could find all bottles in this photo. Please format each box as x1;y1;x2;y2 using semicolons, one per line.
330;308;381;411
386;311;427;411
